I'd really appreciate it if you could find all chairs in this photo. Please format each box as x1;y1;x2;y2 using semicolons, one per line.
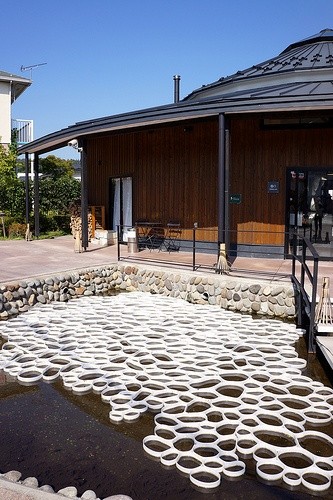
135;227;165;253
